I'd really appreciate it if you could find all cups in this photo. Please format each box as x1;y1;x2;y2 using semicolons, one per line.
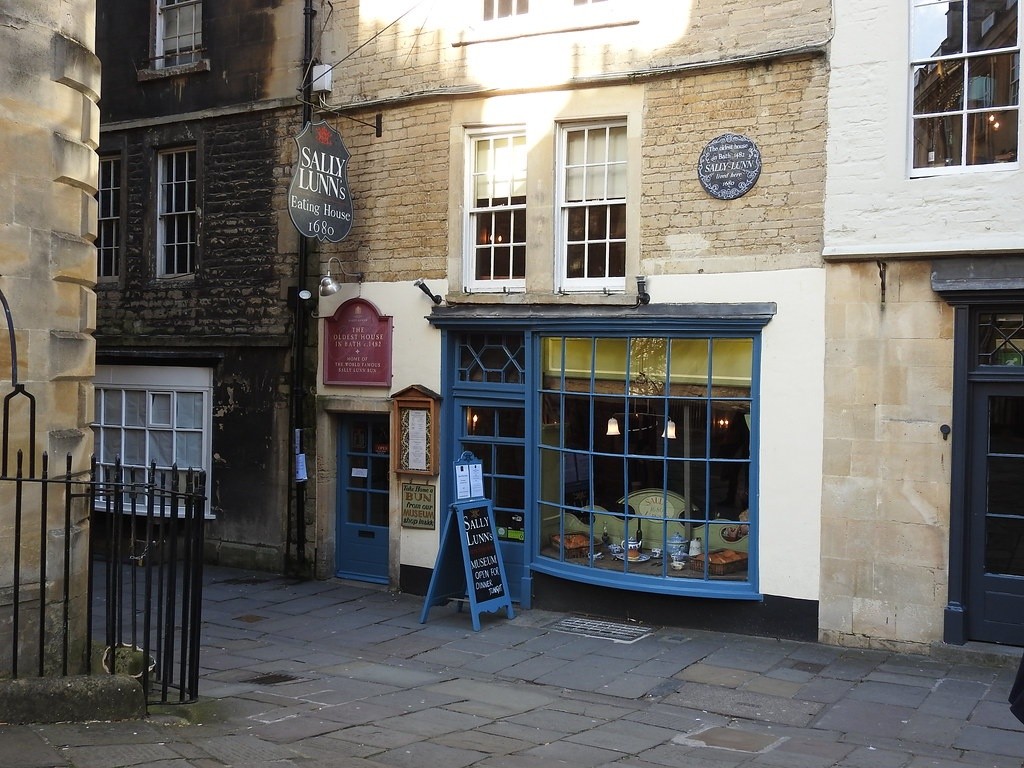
652;548;661;558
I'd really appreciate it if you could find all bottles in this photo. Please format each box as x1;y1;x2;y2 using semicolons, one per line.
602;521;609;547
636;518;642;553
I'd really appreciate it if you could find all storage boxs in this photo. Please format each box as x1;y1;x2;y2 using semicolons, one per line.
549;529;603;558
689;547;748;575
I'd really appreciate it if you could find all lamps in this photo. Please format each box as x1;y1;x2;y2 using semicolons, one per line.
605;337;677;439
318;256;349;297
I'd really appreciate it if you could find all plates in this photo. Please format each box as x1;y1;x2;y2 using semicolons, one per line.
616;553;650;562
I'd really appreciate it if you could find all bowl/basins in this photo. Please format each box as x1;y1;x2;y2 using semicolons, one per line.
671;562;684;570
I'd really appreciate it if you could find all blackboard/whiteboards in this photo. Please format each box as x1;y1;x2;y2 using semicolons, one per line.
452;498;515;632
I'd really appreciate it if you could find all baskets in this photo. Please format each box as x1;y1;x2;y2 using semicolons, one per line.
689;548;748;576
549;531;603;559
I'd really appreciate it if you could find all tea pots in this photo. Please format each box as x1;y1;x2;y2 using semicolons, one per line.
621;537;641;551
